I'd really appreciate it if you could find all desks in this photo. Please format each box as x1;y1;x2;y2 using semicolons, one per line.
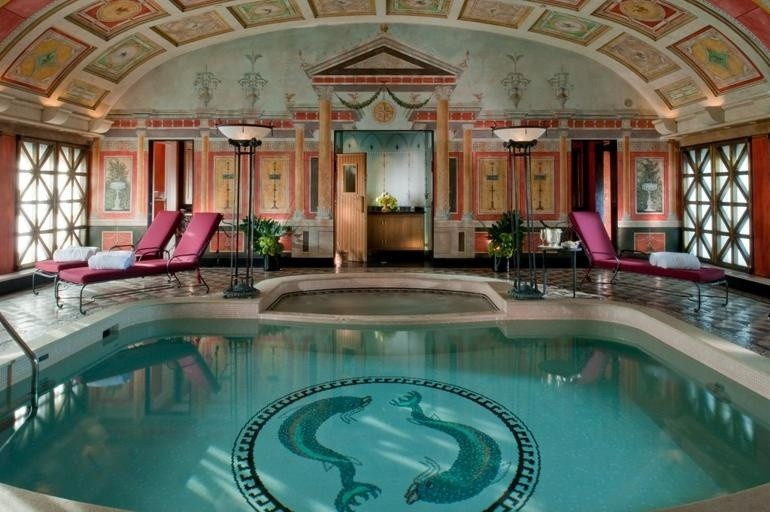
536;242;582;299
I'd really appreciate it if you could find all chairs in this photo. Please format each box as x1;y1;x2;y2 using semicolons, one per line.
32;209;186;298
568;211;729;313
56;212;223;315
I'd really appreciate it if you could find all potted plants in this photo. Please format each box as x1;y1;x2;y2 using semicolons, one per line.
485;210;526;273
240;215;288;272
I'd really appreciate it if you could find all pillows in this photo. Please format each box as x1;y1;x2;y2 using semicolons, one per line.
66;246;100;255
53;248;93;265
88;254;131;270
96;251;135;266
649;250;700;271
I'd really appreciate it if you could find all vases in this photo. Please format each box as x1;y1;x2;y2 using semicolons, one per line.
381;204;391;212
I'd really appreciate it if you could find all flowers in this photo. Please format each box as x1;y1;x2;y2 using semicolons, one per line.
376;191;398;208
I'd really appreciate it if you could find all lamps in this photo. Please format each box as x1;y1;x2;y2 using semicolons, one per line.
489;120;546;299
215;118;273;299
